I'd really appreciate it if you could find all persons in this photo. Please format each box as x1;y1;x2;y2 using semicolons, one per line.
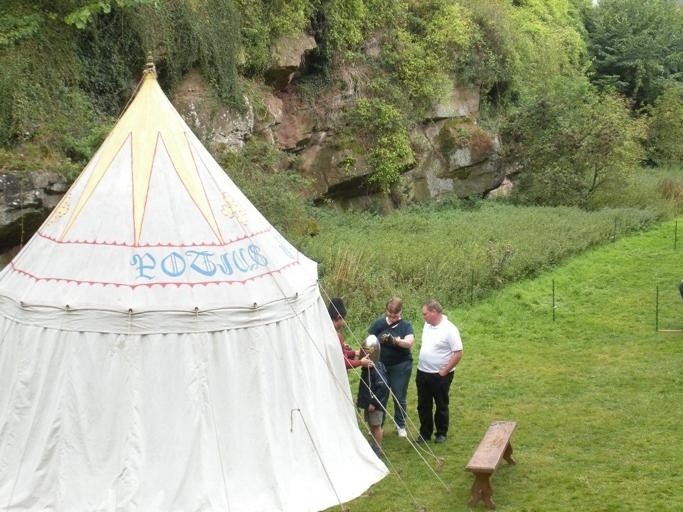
323;297;415;455
416;299;463;444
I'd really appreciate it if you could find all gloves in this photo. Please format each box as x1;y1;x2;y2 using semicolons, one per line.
380;331;401;347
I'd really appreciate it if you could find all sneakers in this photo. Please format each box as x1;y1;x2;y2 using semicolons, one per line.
397;428;447;444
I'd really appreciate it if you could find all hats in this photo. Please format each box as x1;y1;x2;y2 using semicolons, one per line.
328;298;346;314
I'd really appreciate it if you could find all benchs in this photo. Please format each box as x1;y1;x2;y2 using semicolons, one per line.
464;421;516;509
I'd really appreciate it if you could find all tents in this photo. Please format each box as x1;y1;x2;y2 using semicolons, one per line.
0;51;391;512
